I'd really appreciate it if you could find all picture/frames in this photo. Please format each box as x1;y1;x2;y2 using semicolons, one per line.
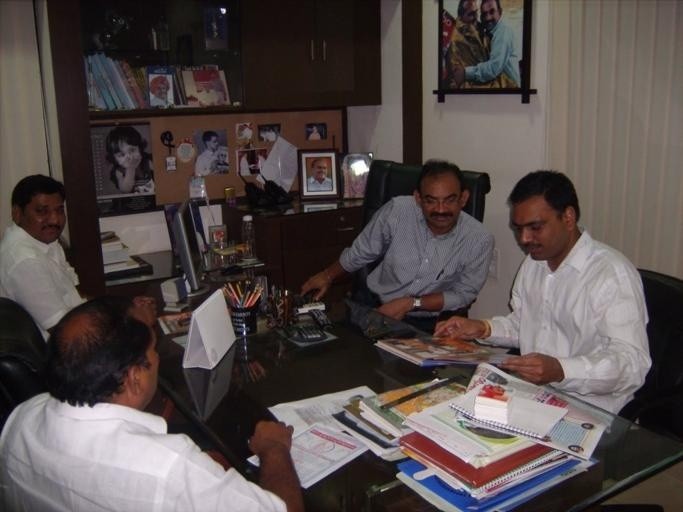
433;1;541;106
297;147;342;200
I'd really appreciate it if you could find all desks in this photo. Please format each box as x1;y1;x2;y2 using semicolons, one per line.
139;293;682;512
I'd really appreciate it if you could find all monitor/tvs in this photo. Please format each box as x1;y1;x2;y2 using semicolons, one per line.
173;199;210;298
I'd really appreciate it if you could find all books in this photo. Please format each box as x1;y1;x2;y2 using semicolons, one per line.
99;230;153;281
373;336;521;367
84;54;231;112
328;375;600;512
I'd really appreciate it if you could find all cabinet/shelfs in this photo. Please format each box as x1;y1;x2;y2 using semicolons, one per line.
226;1;386;114
216;198;363;300
42;1;246;295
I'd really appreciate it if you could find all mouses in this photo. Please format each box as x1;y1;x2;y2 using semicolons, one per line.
222;266;242;276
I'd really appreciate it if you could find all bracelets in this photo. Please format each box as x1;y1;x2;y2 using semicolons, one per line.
479;320;490;338
411;295;421;311
324;269;332;283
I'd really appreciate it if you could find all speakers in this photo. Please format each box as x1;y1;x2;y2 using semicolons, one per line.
204;254;211;271
160;277;190;312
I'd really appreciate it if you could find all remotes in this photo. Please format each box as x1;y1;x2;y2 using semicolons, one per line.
298;301;326;314
309;309;334;330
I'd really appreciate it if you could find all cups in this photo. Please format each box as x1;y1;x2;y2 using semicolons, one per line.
230;306;258;336
234;336;257;364
223;187;236;209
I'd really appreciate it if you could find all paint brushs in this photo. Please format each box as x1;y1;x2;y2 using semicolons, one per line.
380;374;468;410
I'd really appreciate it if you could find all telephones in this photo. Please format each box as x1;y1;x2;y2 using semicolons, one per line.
238;171;290;206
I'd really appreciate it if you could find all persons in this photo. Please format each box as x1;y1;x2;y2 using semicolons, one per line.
305;159;331;192
306;125;319;139
262;127;276;143
445;0;516;89
433;170;654;428
104;127;154;195
194;131;227;175
236;123;252;150
297;161;493;332
253;152;265;172
213;149;227;173
453;0;521;89
238;152;251;177
0;174;158;346
0;296;310;512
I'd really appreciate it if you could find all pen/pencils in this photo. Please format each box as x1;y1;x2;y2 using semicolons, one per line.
223;280;264;308
268;284;288;327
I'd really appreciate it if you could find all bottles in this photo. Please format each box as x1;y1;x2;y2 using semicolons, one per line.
200;224;241;271
241;214;257;266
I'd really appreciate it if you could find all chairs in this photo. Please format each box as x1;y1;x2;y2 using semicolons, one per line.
0;295;73;399
0;355;46;420
616;270;682;423
316;155;491;304
